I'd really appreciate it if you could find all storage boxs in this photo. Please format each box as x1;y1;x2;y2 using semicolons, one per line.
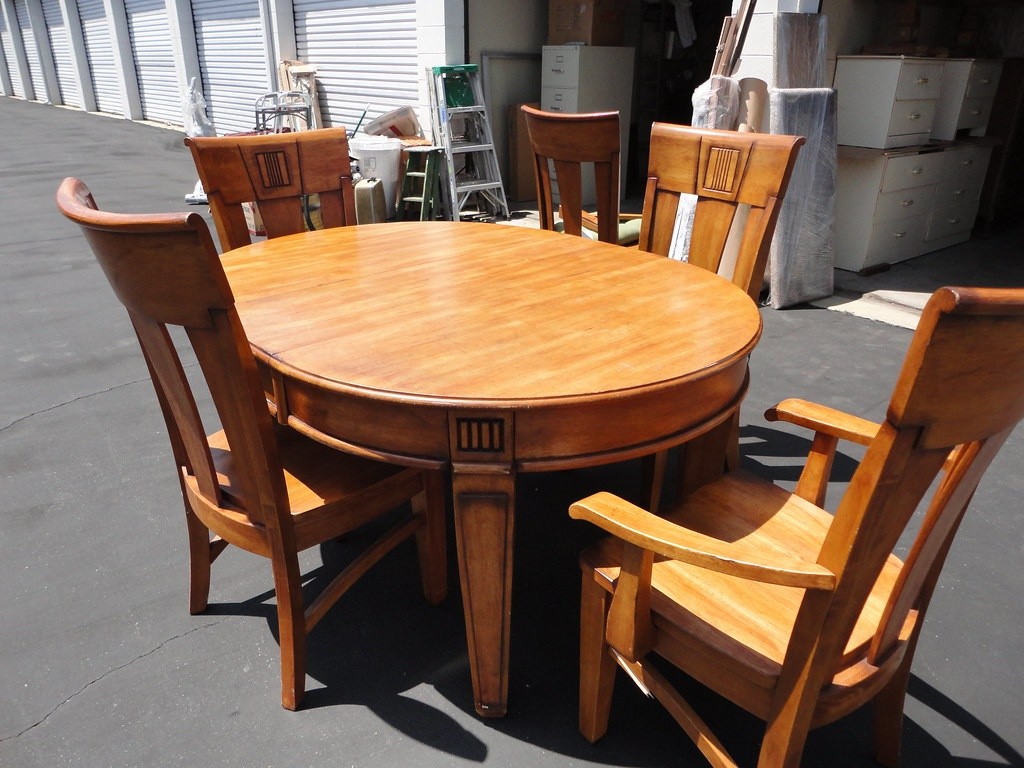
547;0;627;46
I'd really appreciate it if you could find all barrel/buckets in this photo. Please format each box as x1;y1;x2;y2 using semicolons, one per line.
350;137;402;220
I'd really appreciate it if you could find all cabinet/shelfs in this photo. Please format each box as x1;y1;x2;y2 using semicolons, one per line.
829;55;948;150
538;45;638;208
830;140;999;273
930;56;1006;143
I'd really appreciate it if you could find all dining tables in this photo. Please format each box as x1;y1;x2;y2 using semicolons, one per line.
215;220;765;722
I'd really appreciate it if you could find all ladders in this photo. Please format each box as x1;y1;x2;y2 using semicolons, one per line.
425;63;511;216
394;146;445;221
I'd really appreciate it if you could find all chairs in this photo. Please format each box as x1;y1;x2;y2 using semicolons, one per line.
637;120;809;476
567;285;1023;768
521;102;645;248
184;126;359;252
56;176;453;711
